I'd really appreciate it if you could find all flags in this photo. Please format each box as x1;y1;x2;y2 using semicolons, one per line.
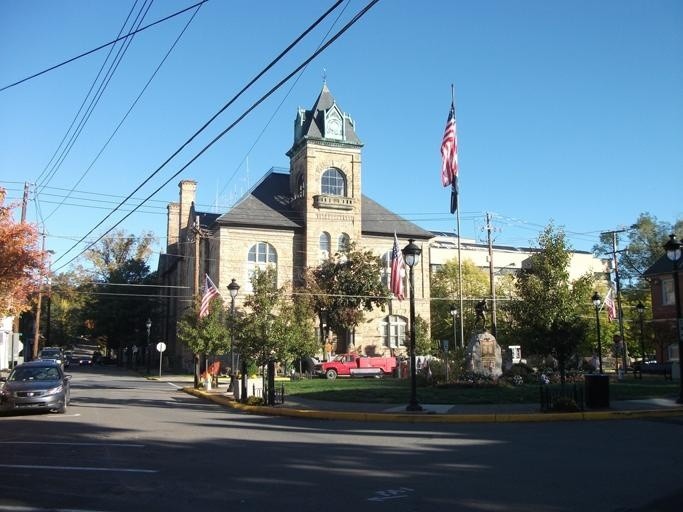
199;276;218;318
391;239;405;302
440;105;458;213
604;290;616;322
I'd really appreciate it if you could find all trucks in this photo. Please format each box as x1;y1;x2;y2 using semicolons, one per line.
316;354;397;379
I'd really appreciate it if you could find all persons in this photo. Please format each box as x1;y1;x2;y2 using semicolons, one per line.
474;299;489;329
322;339;333;362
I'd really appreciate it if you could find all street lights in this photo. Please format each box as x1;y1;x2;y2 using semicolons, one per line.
592;291;603;374
636;302;646;363
145;318;152;376
227;279;240;392
401;239;422;411
664;234;683;403
449;304;458;351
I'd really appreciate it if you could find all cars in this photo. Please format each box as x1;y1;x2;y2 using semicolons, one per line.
37;347;70;372
79;356;92;365
1;362;70;414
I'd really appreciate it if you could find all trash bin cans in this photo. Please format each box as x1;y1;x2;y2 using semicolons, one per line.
584;374;609;408
618;369;625;380
